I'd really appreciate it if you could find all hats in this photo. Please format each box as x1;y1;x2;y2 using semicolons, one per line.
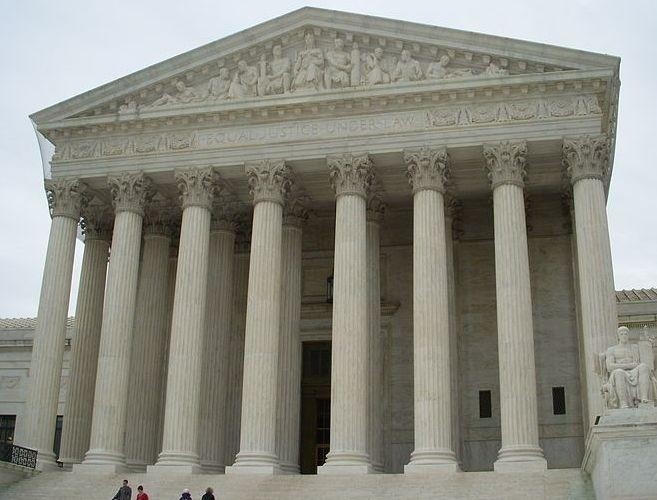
183;489;189;494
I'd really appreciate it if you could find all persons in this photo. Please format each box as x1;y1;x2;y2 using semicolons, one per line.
201;487;215;500
130;29;448;108
111;480;131;500
604;323;657;408
178;489;192;500
136;486;148;500
